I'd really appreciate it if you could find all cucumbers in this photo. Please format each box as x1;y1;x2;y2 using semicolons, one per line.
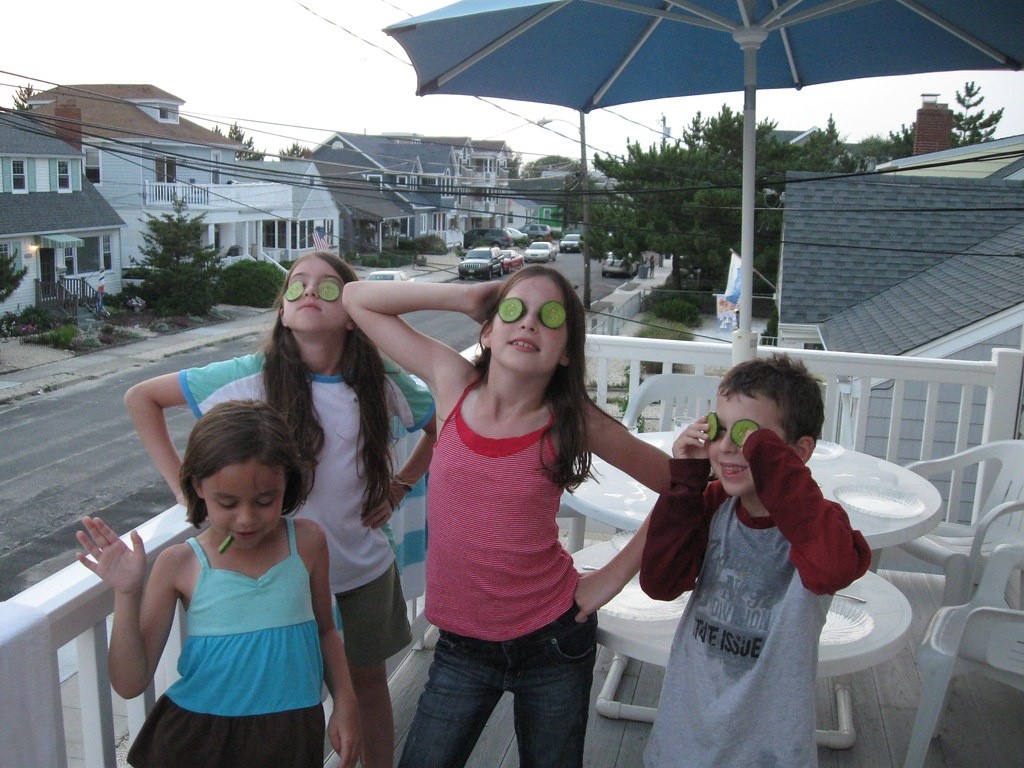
218;535;235;555
498;297;525;323
318;281;340;301
731;419;759;446
285;280;305;302
705;412;720;442
541;301;566;329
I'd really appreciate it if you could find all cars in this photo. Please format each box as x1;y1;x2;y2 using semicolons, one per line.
500;250;529;274
603;251;641;278
365;270;414;282
559;233;584;253
524;242;557;262
507;228;529;241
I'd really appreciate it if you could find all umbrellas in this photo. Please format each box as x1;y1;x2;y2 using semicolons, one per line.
380;0;1024;333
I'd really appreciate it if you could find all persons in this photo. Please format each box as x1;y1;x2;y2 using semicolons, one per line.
123;253;440;768
343;265;674;768
649;255;655;279
639;352;872;768
76;399;361;768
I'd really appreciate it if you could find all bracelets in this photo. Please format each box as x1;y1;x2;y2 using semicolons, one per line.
395;475;412;491
388;497;394;512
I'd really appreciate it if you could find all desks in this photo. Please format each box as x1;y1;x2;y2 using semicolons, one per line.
556;430;943;750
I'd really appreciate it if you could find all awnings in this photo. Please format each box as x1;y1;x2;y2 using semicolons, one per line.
33;234;85;248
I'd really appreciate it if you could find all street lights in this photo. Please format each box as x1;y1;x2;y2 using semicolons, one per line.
537;117;601;313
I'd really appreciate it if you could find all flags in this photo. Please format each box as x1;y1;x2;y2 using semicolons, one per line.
311;227;329;255
95;267;105;315
724;253;742;306
716;294;741;333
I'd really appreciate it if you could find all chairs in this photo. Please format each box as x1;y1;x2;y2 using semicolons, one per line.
574;373;1024;768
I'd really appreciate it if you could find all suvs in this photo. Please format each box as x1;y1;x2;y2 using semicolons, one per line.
519;224;555;240
458;246;505;280
463;227;513;248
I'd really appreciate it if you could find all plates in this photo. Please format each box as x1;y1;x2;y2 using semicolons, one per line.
599;575;695;621
813;440;846;460
612;528;636;551
833;484;926;519
819;601;876;645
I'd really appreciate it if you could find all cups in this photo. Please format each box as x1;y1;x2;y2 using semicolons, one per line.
673;416;695;441
626;426;638;439
